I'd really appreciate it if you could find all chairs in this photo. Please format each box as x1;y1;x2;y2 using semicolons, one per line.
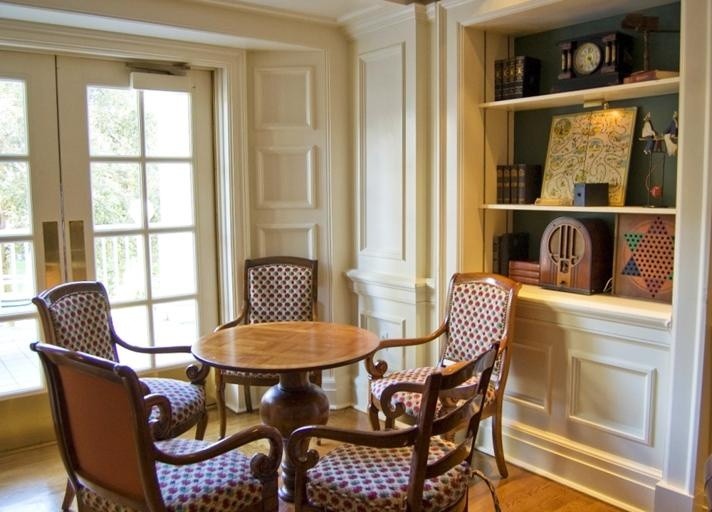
287;339;500;512
214;257;323;443
34;281;209;511
366;272;522;479
31;341;283;510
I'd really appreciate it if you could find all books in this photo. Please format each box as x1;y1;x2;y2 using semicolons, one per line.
494;55;541;101
496;164;503;204
492;230;541;285
510;164;518;204
503;164;510;203
517;162;541;205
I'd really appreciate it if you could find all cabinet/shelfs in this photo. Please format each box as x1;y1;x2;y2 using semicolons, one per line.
445;1;712;512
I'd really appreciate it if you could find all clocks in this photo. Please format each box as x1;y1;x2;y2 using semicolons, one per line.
556;31;636;92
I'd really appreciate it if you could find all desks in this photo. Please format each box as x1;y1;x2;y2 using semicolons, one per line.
191;322;380;501
